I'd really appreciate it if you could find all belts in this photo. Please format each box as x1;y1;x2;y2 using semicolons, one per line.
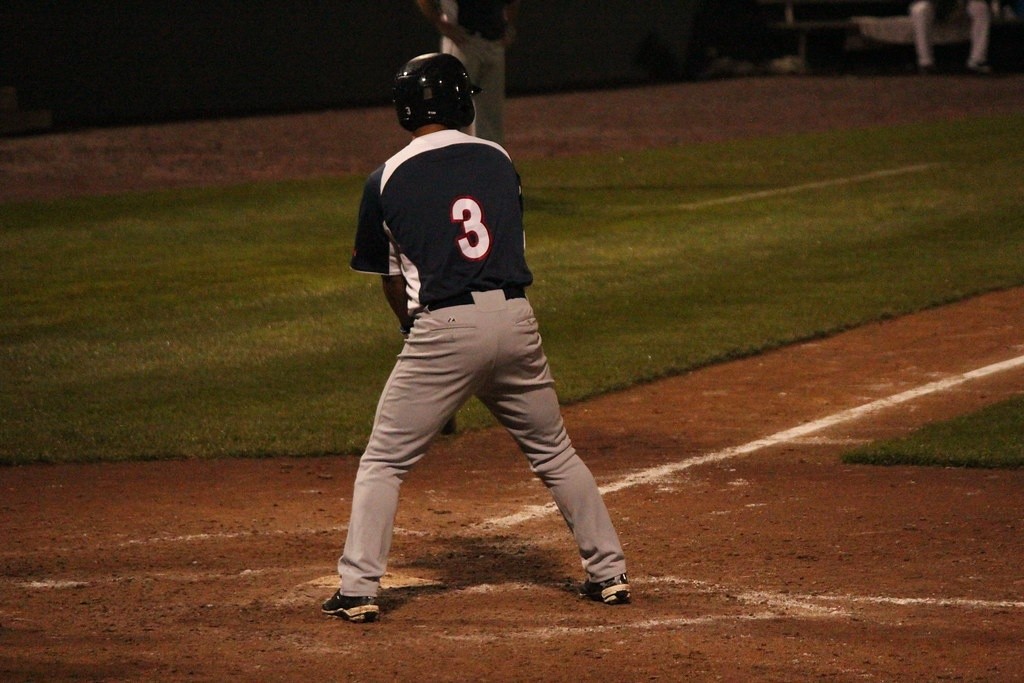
428;286;526;312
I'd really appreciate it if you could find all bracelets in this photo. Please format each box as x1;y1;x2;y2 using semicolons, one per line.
399;326;408;336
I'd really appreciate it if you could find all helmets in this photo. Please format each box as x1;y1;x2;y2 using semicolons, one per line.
394;52;483;130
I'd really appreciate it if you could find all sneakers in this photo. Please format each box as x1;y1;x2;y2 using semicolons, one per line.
321;588;379;621
580;572;631;604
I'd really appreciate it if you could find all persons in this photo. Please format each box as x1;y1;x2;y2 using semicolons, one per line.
908;0;993;74
418;0;518;144
319;52;632;621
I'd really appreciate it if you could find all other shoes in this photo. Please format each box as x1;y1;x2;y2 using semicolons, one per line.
920;66;939;75
967;62;989;80
